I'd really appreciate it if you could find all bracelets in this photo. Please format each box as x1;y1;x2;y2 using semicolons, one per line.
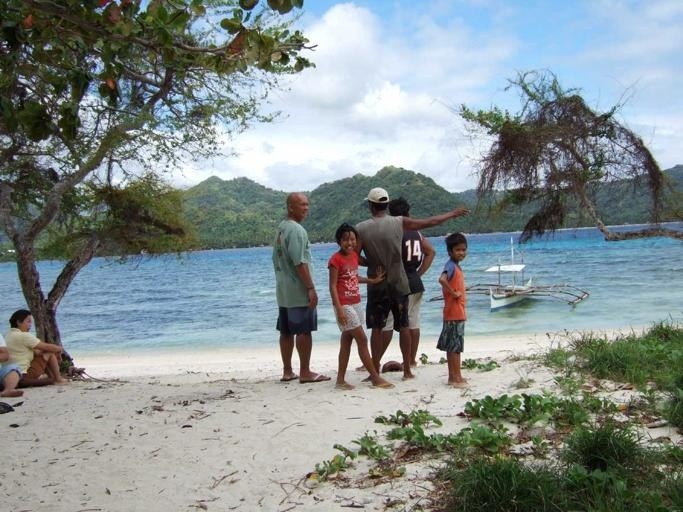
308;287;315;290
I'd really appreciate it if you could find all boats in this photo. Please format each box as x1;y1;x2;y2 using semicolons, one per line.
482;234;534;315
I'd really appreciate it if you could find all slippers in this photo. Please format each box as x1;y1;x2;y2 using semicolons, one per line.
300;373;332;384
335;382;356;390
374;380;396;390
280;373;299;382
363;187;390;205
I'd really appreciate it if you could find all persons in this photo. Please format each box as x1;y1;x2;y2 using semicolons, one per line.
272;192;331;383
328;222;396;390
355;197;435;371
436;233;477;388
0;335;24;397
354;187;469;382
1;310;73;388
